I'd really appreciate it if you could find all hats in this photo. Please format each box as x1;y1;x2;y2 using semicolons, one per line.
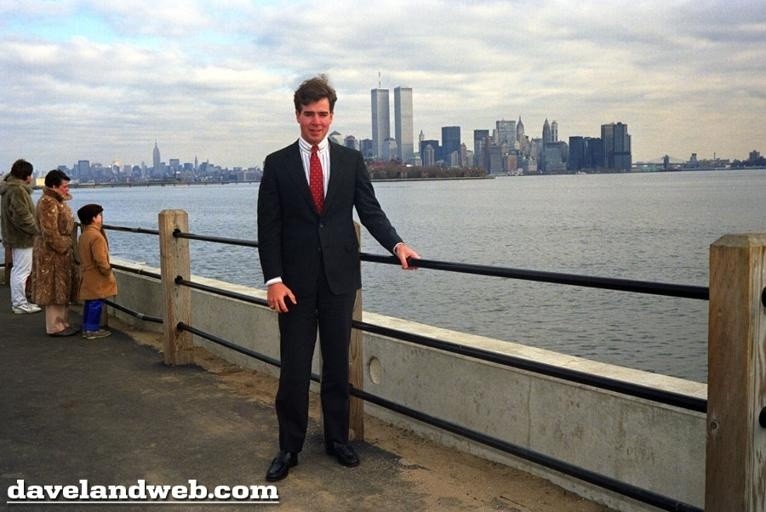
76;204;103;224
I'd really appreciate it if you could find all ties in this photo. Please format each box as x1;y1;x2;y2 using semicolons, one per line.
308;144;325;216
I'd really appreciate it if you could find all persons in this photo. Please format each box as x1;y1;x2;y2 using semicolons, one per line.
76;204;117;339
31;169;81;337
258;71;420;483
0;158;43;315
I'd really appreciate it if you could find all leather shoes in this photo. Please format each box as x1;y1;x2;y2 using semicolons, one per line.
11;302;43;316
265;449;298;483
324;438;361;467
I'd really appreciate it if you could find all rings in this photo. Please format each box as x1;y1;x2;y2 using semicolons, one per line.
270;304;274;309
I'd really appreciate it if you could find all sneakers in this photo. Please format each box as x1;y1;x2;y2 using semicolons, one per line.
48;326;82;337
82;329;111;341
